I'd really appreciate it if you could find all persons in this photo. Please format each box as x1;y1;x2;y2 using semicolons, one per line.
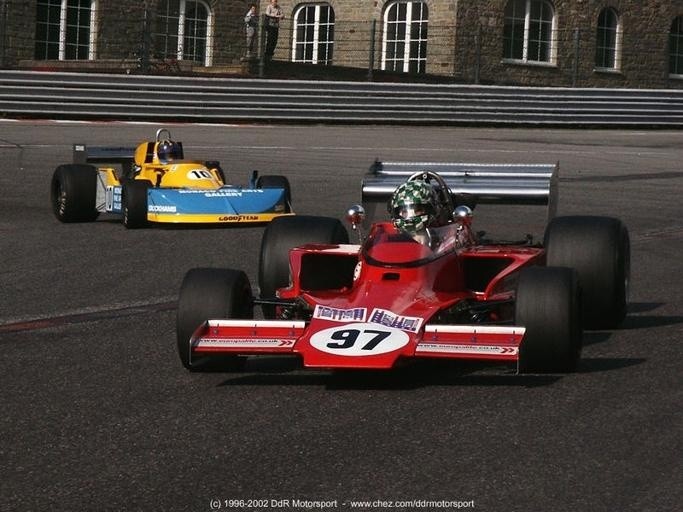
158;140;180;162
382;177;446;253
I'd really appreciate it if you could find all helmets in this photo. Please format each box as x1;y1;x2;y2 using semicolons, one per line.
156;139;181;162
386;179;440;232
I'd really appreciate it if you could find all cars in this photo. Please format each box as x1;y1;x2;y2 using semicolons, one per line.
50;127;295;228
